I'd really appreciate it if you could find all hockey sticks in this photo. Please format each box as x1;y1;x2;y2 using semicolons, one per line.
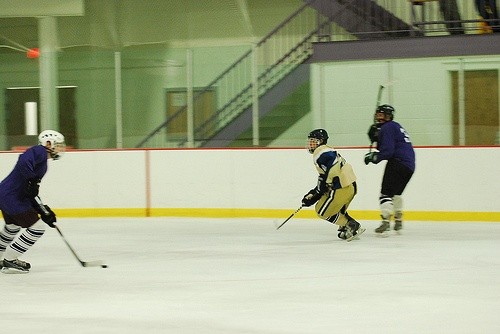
369;84;384;153
276;203;305;230
35;196;104;268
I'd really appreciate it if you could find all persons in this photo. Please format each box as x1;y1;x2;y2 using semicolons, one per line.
364;104;415;233
301;129;361;239
0;130;66;271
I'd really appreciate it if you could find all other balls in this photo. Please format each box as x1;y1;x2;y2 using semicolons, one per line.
102;265;107;268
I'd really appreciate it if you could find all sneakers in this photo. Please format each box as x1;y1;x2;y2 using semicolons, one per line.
375;220;391;238
338;219;366;242
1;259;31;274
393;219;404;235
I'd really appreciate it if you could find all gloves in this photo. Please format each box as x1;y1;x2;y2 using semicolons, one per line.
37;205;56;228
364;152;373;165
302;186;324;207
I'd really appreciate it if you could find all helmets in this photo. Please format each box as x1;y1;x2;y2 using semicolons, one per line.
376;105;395;115
308;129;329;141
38;130;64;146
